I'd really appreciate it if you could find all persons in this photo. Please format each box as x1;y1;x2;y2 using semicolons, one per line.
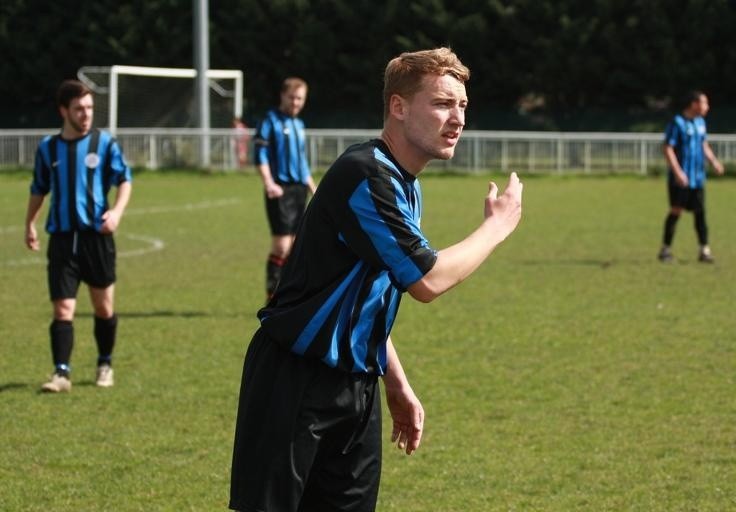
227;46;524;512
657;93;725;265
252;76;319;304
25;80;132;394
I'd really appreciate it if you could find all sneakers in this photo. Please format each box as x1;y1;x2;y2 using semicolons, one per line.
95;363;114;388
41;374;71;393
657;248;677;264
698;253;716;264
